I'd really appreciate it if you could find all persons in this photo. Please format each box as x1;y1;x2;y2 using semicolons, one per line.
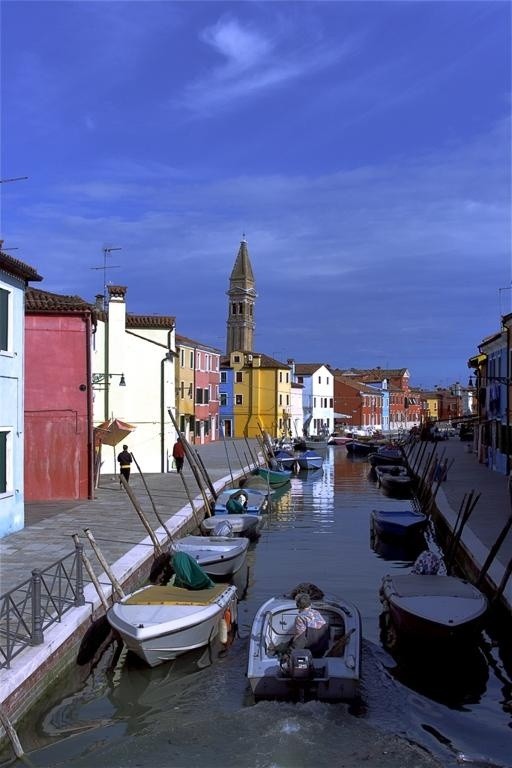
117;445;133;490
284;594;326;660
173;438;185;473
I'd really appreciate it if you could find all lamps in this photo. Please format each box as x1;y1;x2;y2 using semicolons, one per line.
465;372;510;390
91;371;127;390
